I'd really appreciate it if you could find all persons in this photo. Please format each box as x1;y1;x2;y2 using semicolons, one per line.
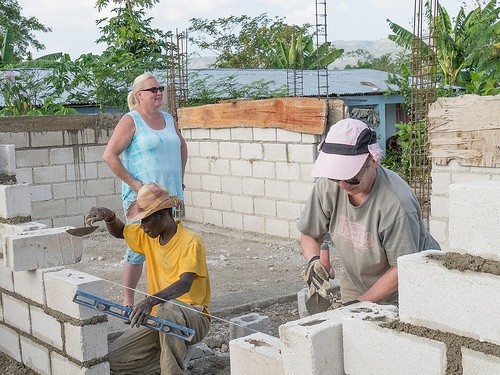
101;72;189;325
295;117;442;311
85;183;211;375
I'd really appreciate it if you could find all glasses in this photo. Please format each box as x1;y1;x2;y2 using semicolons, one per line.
139;85;164;93
328;166;367;185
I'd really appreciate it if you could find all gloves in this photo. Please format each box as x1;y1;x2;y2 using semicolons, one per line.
304;256;335;292
327;300;361;313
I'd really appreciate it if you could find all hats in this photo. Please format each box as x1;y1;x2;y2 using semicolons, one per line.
124;182;179;222
311;118;383;180
